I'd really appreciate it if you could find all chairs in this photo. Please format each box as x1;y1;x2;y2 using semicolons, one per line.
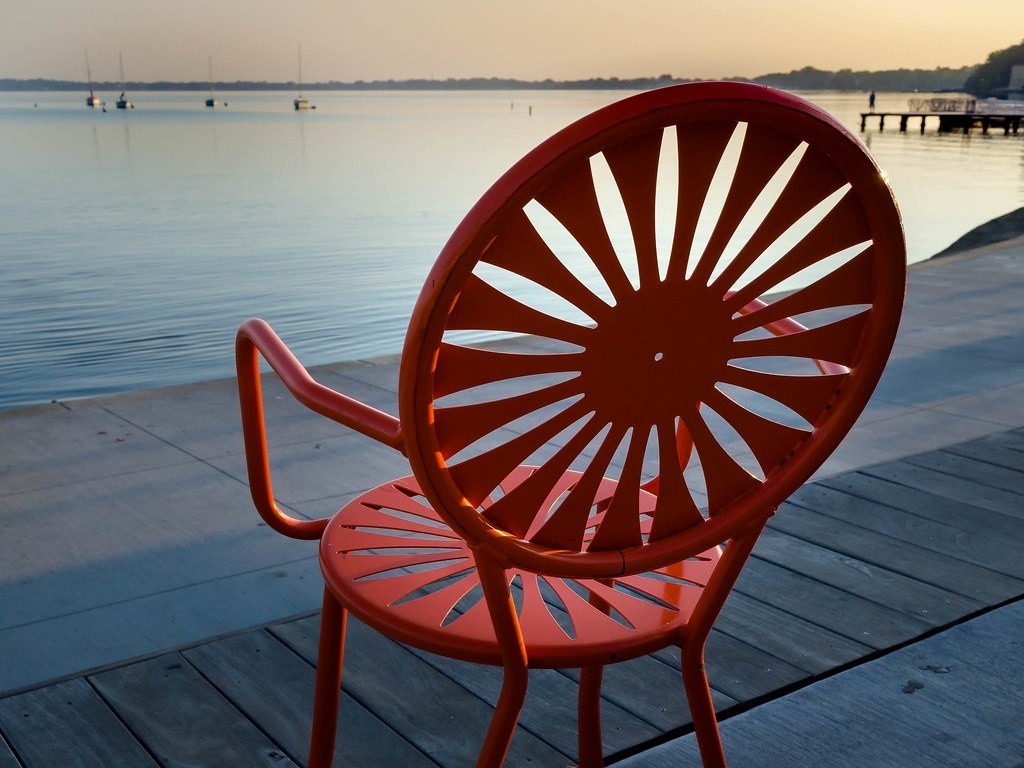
235;82;907;768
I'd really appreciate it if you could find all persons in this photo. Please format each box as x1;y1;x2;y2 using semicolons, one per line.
869;92;875;113
